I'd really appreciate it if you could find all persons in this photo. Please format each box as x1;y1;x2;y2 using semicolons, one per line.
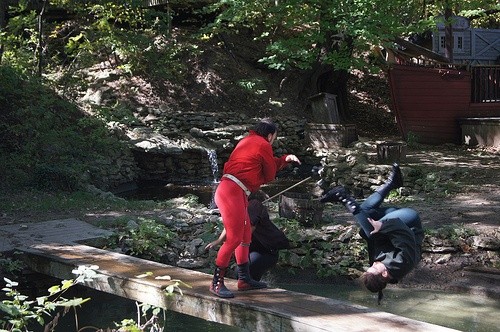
203;190;291;283
318;162;426;305
207;121;302;299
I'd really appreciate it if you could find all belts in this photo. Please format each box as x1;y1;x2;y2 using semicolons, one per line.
220;173;252;197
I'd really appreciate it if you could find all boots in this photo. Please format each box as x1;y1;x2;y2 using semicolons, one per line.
208;264;235;300
236;261;268;291
375;162;403;198
321;185;362;215
